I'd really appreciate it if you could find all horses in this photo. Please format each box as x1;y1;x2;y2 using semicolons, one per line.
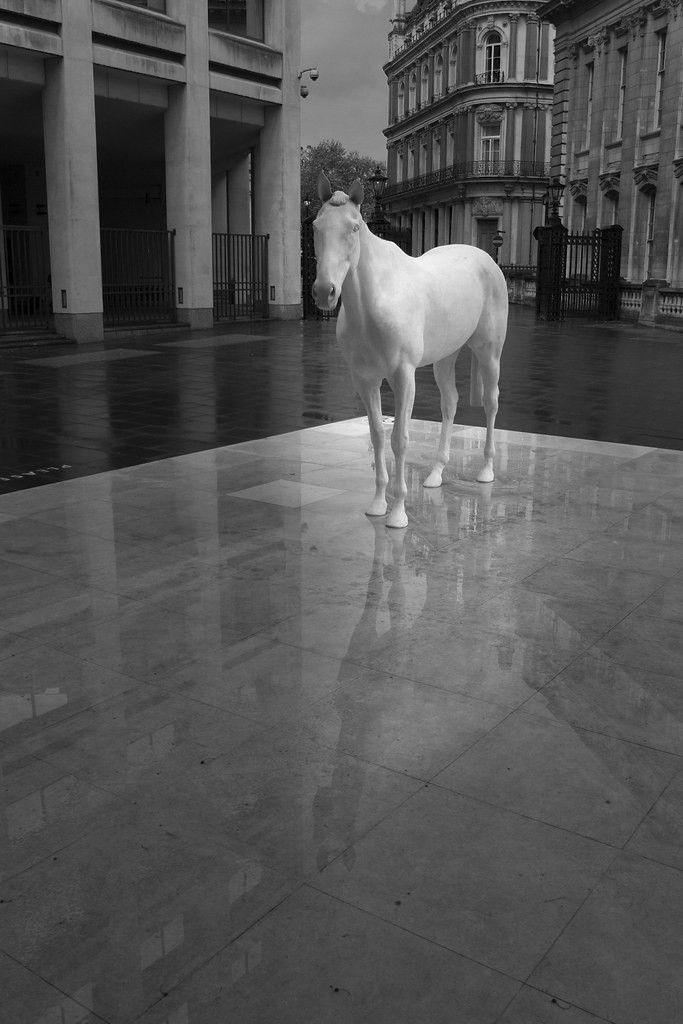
311;169;509;528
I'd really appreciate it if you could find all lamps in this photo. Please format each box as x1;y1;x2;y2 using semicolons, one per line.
546;178;565;220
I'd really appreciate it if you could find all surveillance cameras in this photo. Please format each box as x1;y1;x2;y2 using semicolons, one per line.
310;69;318;81
300;87;309;98
